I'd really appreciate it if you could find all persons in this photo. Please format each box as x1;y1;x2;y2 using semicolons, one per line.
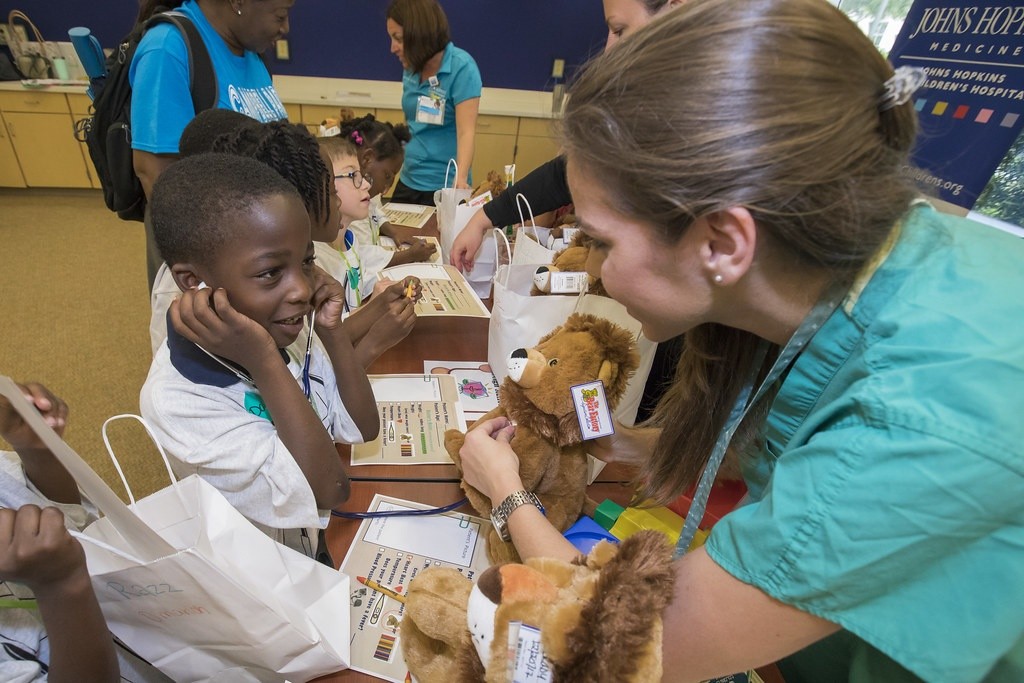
385;0;482;209
322;114;437;279
449;0;690;427
139;155;381;571
129;0;294;302
210;118;423;381
459;0;1024;683
308;137;401;326
150;109;261;371
0;383;175;683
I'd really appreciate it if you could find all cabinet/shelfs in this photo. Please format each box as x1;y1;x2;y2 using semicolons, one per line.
0;74;571;190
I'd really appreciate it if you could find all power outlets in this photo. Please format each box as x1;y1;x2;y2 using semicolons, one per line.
276;39;288;61
552;59;565;77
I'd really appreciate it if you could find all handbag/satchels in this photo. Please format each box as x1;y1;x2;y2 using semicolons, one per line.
24;412;352;683
511;193;575;264
433;158;517;299
488;227;659;485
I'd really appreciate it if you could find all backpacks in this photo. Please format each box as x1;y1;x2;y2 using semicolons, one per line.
72;11;216;222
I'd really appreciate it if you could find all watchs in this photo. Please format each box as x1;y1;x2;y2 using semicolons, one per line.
490;492;546;544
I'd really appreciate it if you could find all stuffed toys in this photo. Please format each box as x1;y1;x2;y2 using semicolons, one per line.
525;204;585;253
399;530;676;683
530;230;613;300
443;313;637;565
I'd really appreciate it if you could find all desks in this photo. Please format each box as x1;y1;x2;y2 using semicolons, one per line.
303;482;782;683
336;204;636;481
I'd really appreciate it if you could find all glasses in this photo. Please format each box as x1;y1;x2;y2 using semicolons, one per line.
333;170;374;190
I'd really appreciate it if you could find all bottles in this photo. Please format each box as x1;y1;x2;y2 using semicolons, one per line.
52;56;69;79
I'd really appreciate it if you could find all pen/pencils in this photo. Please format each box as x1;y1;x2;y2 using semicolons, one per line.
406;279;413;307
